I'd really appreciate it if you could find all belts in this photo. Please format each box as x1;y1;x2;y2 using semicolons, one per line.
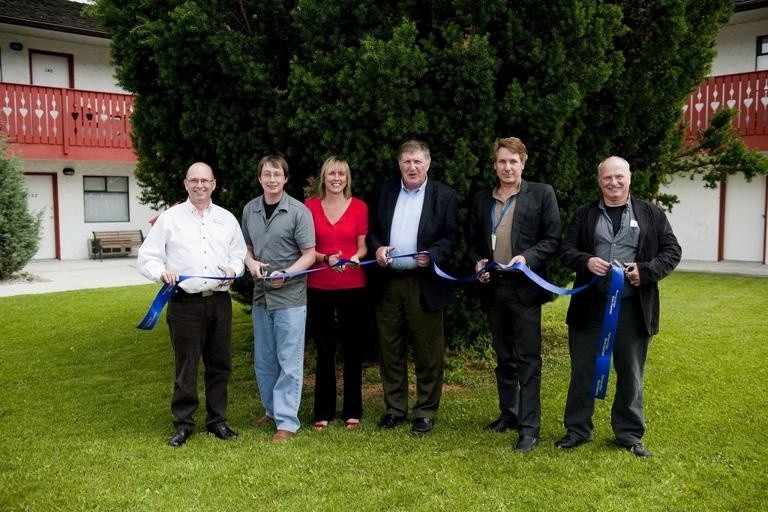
180;290;228;297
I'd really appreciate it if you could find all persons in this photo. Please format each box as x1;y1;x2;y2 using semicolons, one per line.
303;155;368;431
137;163;247;447
556;156;682;457
242;153;316;443
364;139;461;434
465;137;561;453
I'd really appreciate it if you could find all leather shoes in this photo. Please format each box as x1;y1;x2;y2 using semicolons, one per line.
555;433;587;449
207;424;239;440
516;434;539;452
168;430;188;446
252;415;271;426
379;413;407;428
615;437;650;458
485;416;508;432
272;431;293;442
412;417;434;432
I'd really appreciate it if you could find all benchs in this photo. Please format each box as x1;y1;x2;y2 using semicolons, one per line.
92;230;145;263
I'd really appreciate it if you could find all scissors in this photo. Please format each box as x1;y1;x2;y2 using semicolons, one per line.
478;259;485;281
613;259;633;280
261;265;268;280
386;247;390;265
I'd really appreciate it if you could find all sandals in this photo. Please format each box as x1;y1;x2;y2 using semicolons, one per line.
313;421;328;430
345;418;359;429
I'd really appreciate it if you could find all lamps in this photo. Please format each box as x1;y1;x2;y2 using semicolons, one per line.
63;169;74;175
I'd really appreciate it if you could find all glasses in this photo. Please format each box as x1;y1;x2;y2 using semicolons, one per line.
186;177;214;185
261;172;286;179
